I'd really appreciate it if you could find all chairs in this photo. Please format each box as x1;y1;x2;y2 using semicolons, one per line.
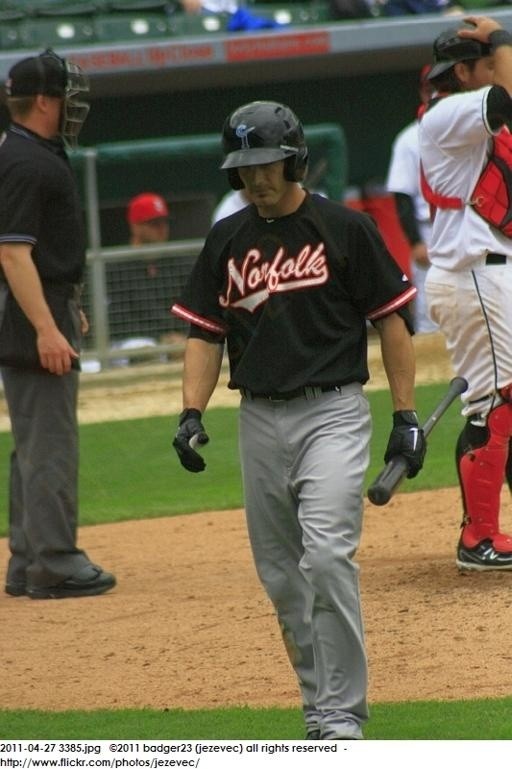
1;0;333;55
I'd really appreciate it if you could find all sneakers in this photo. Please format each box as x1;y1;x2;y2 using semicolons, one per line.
25;566;120;600
454;530;512;574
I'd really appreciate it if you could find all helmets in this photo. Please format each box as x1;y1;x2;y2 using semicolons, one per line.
219;100;308;191
424;22;492;94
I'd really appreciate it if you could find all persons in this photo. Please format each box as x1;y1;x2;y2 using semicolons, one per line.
385;63;443;335
210;183;328;231
417;14;511;571
172;99;427;740
127;192;193;319
0;48;115;600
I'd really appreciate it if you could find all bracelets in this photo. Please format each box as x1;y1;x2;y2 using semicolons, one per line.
487;30;511;55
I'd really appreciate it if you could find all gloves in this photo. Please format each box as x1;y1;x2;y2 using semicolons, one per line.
382;409;427;480
171;406;208;475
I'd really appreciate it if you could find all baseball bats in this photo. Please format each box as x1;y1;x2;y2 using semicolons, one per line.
369;378;468;505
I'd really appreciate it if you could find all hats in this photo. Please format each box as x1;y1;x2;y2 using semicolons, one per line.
126;193;176;225
6;54;67;99
